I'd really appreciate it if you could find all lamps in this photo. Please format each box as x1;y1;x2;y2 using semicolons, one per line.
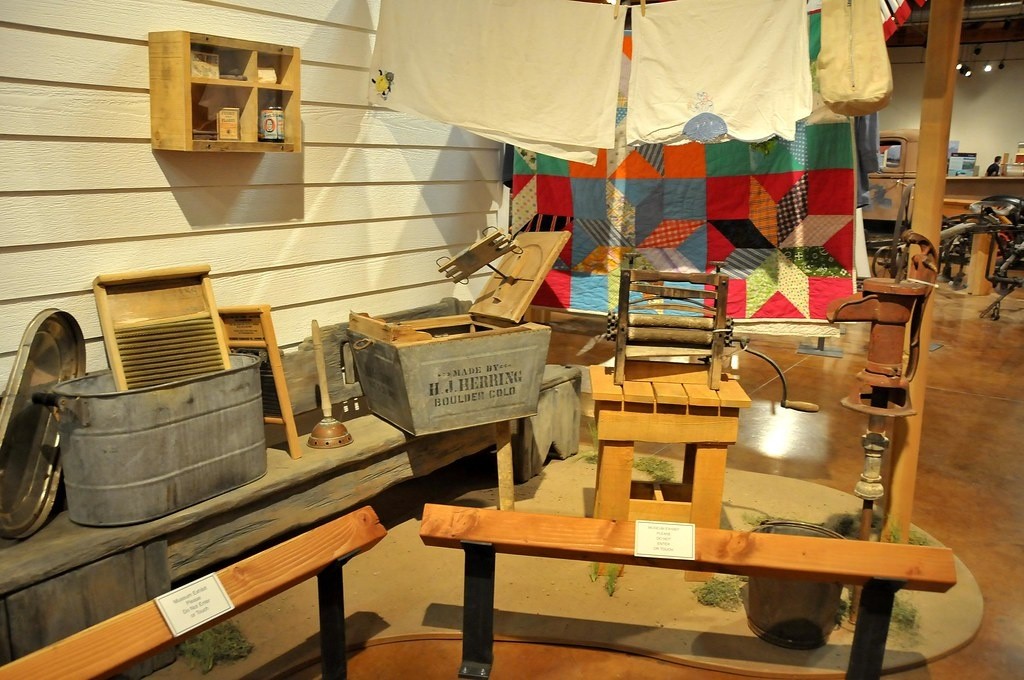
957;43;1008;77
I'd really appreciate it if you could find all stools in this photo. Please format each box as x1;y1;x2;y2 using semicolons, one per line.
590;363;753;585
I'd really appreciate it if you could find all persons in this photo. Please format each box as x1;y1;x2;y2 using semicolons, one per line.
985;156;1002;176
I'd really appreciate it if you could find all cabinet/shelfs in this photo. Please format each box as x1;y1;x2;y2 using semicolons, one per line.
865;173;1024;223
148;29;302;154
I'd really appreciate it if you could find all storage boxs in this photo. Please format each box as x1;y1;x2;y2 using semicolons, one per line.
348;311;553;438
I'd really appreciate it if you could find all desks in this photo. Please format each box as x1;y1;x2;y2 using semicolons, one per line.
0;419;514;680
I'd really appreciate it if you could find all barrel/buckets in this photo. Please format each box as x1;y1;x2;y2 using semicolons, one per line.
32;348;268;529
747;520;848;649
